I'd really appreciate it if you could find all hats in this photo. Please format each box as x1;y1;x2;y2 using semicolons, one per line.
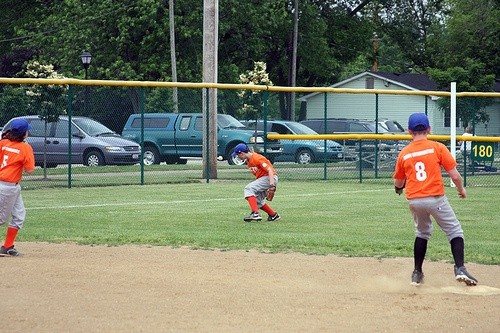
409;112;430;132
10;119;32;134
232;143;248;156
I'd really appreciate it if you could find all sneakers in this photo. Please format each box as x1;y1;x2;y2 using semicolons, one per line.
268;212;280;222
243;211;262;221
412;270;424;286
0;245;22;256
453;265;478;285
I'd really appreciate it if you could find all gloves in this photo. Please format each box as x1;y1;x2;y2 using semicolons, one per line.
394;183;406;195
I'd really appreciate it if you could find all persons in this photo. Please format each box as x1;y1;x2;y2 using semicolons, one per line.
231;143;280;223
461;125;477;168
0;119;35;257
392;113;479;286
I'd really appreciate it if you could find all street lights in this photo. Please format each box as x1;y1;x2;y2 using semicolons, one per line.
81;51;92;116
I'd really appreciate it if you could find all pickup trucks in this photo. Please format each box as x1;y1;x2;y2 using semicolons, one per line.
122;113;284;166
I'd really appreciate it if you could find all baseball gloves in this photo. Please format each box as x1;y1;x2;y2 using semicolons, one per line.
265;184;275;202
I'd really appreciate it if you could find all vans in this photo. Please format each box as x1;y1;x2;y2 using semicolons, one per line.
1;115;146;169
299;118;410;161
238;120;346;164
